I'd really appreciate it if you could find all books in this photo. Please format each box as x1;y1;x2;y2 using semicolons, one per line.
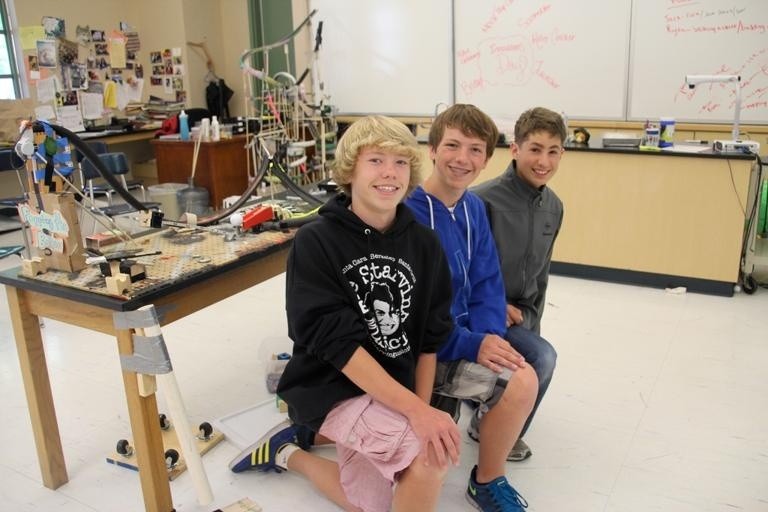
147;101;184;122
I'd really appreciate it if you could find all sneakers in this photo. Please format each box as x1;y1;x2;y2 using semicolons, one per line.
467;407;532;462
228;417;298;474
465;464;528;512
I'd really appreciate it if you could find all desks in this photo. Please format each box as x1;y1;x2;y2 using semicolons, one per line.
0;182;354;511
82;119;315;213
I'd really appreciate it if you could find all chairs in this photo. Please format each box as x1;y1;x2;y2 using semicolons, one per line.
155;108;212;139
0;142;163;245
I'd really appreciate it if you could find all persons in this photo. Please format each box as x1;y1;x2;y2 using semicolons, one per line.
227;113;461;512
461;107;568;462
294;103;540;512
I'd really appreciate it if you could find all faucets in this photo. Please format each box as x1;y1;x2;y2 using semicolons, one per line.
434;102;448;120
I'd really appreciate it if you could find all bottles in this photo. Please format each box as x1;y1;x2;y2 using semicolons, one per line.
646;121;674;148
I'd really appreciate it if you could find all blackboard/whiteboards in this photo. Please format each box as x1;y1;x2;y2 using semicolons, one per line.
454;0;633;121
627;0;768;124
306;2;454;119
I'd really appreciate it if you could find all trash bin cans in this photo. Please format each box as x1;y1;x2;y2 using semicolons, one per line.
148;183;188;222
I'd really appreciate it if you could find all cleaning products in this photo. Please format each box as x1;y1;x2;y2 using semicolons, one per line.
179;109;190;140
